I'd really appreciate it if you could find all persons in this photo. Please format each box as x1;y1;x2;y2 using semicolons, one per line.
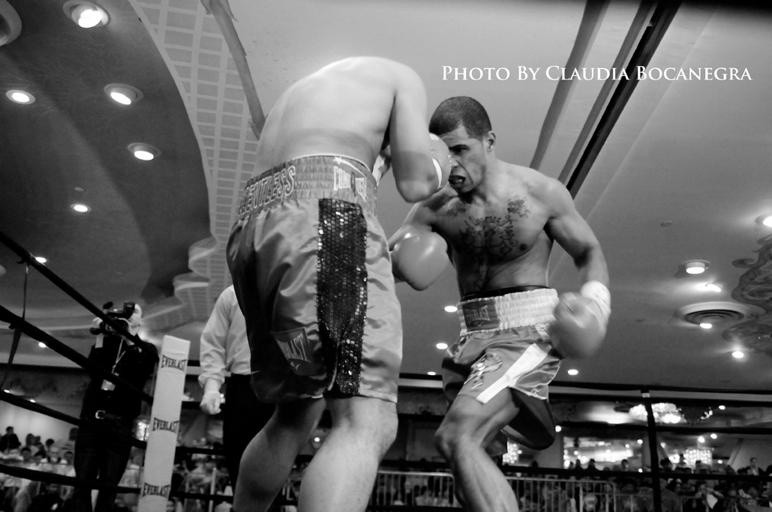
385;95;612;510
225;54;452;512
0;422;771;510
195;284;287;510
66;298;159;510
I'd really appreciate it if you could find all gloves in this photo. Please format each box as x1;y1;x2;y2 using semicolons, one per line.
200;379;222;415
392;230;451;291
547;291;608;359
429;131;452;194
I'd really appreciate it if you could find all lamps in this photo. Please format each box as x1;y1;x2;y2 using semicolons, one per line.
1;0;159;214
426;305;458;376
676;215;771;360
